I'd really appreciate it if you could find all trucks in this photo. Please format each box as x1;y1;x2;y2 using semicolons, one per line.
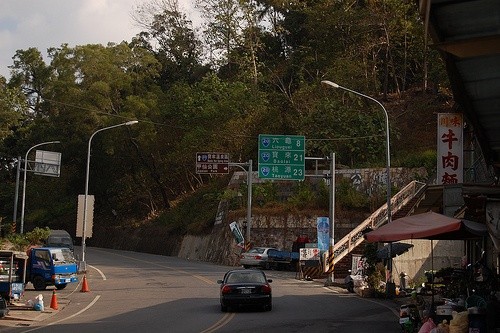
0;250;28;307
15;247;79;292
267;241;306;272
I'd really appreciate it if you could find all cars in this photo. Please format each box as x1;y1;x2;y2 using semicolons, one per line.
240;246;278;270
217;269;273;313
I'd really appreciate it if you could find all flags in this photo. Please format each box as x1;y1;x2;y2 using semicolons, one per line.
230;222;244;247
317;217;329;255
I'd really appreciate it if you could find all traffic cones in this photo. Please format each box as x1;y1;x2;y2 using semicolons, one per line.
50;288;58;310
79;275;90;292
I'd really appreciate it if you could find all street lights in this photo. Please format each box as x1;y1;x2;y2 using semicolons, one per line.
78;120;139;275
320;79;392;284
20;141;61;234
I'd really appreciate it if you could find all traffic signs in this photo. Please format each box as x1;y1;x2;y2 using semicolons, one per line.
196;152;229;175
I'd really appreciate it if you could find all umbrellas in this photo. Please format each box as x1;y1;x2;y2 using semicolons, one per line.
375;243;414;288
363;210;487;312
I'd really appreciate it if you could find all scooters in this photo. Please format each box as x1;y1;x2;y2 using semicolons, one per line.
399;282;429;333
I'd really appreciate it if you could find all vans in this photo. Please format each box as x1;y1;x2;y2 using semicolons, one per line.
42;230;74;256
